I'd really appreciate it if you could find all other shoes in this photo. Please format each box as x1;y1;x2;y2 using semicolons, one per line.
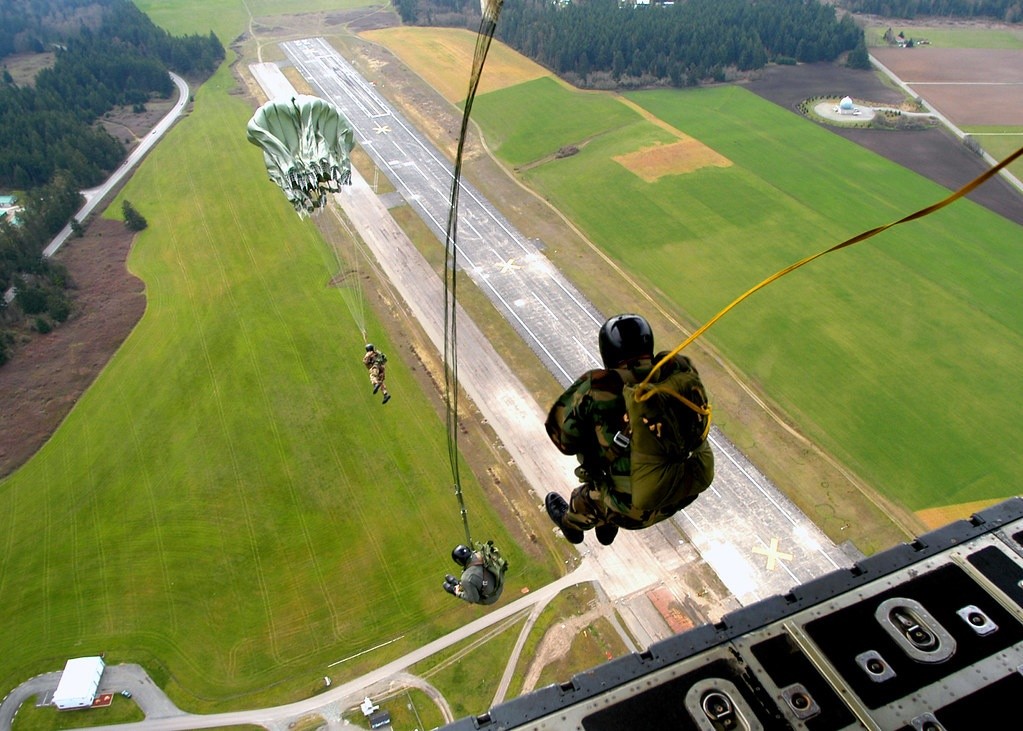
382;395;391;404
373;383;381;394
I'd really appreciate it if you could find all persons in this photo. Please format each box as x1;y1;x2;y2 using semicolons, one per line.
443;545;504;605
362;344;390;404
545;313;713;546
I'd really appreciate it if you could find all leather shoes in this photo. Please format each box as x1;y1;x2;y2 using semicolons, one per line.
442;582;456;596
545;492;584;544
445;575;458;586
595;525;619;546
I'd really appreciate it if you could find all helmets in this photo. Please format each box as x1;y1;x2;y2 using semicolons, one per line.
598;313;654;368
365;344;374;351
452;544;472;566
